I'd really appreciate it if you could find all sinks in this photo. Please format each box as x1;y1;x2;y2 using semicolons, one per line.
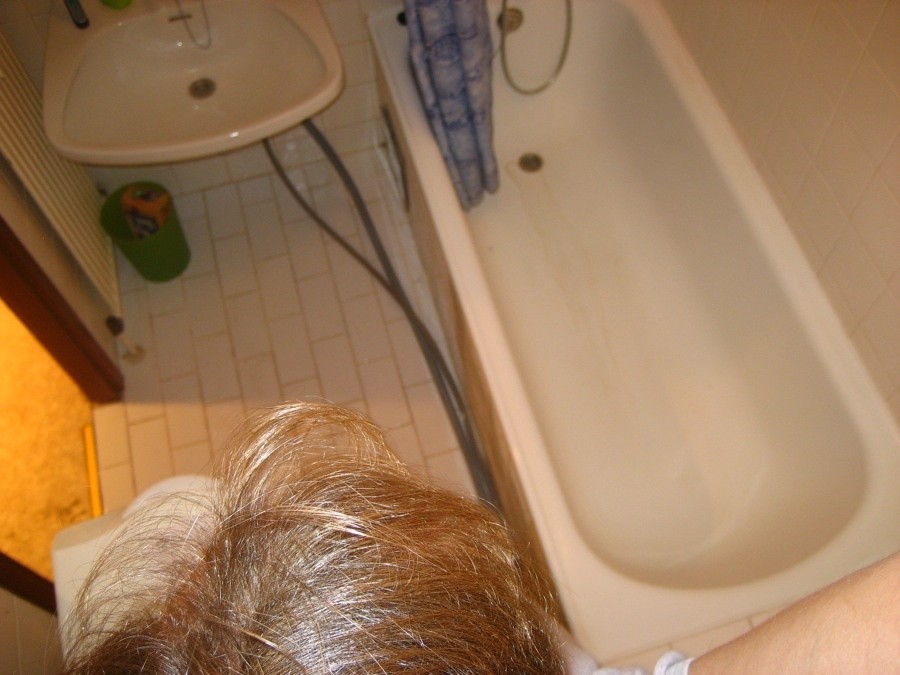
41;0;348;166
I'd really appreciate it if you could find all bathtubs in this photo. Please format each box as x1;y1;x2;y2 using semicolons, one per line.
365;0;900;675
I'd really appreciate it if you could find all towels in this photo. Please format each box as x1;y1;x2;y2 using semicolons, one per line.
401;0;499;211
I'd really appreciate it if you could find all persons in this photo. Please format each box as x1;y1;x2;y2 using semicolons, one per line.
52;397;900;675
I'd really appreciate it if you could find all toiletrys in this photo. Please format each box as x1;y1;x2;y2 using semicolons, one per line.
62;0;89;29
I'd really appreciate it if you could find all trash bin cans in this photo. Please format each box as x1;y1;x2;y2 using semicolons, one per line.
99;179;192;283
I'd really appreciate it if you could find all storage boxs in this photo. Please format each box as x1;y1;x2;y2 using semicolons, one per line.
122;184;171;240
